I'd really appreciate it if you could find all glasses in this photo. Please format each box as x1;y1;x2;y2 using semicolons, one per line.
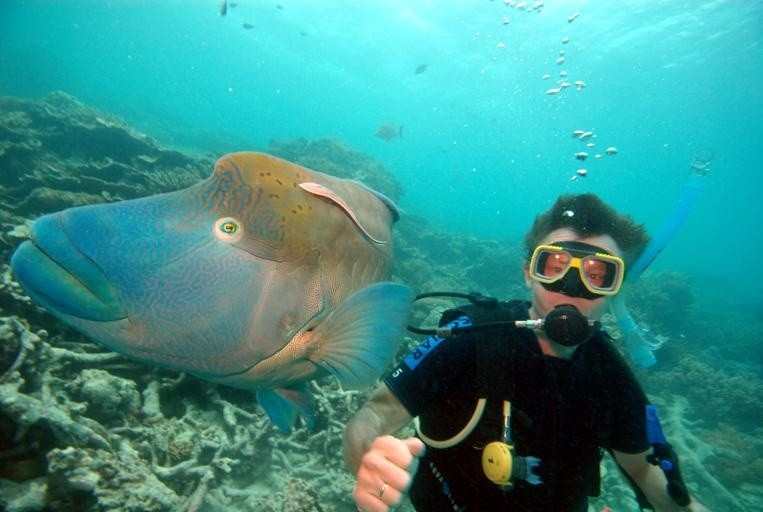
528;241;625;300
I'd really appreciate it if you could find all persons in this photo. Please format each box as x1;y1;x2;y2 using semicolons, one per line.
342;193;694;512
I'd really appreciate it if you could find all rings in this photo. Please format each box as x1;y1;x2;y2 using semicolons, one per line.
379;484;387;497
